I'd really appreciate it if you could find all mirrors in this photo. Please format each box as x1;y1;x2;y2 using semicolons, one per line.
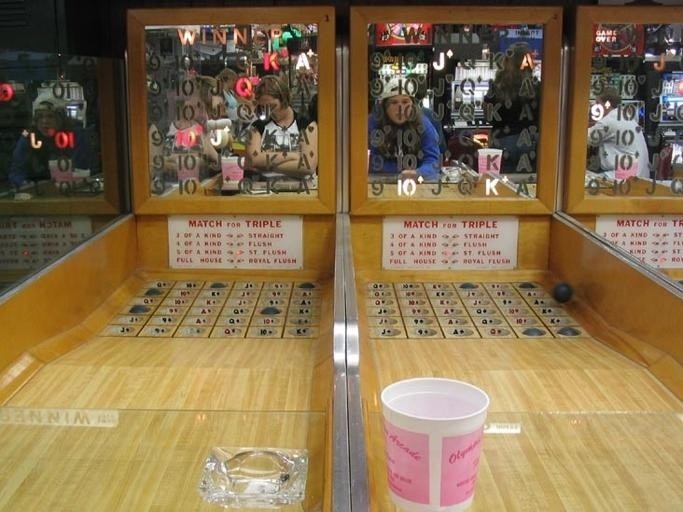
349;5;563;214
564;4;683;215
0;58;124;214
126;6;337;216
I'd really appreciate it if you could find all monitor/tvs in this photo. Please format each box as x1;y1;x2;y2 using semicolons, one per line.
65;100;87;120
451;81;491;125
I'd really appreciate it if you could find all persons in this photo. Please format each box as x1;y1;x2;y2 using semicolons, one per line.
244;75;318;184
586;87;649;182
481;42;541;175
366;76;440;184
162;96;219;181
405;73;448;162
8;90;102;188
207;67;241;137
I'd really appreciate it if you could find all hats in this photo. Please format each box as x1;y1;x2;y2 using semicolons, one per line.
32;93;66;117
379;78;414;101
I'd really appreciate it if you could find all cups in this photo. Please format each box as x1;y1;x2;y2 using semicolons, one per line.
50;159;72;188
222;157;245;191
478;149;502;174
614;152;639;181
175;153;199;184
381;377;490;512
